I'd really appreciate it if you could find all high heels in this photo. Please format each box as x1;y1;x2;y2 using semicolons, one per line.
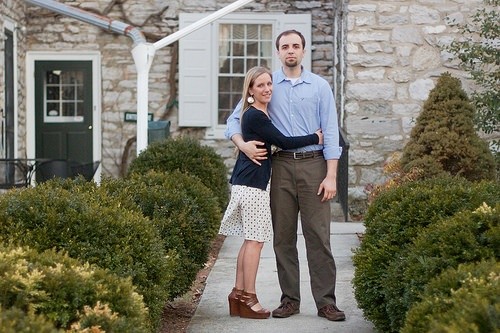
228;287;243;316
239;291;271;319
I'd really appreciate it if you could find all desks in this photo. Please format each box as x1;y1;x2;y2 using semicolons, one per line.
0;158;67;188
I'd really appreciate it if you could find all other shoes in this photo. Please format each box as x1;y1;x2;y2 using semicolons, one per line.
272;301;301;318
317;303;346;320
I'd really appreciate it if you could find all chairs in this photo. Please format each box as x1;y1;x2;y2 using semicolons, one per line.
71;160;102;183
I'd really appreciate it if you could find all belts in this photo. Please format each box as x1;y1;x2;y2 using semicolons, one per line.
273;150;323;160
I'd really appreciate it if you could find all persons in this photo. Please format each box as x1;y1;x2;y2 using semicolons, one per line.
217;67;323;320
224;28;345;321
